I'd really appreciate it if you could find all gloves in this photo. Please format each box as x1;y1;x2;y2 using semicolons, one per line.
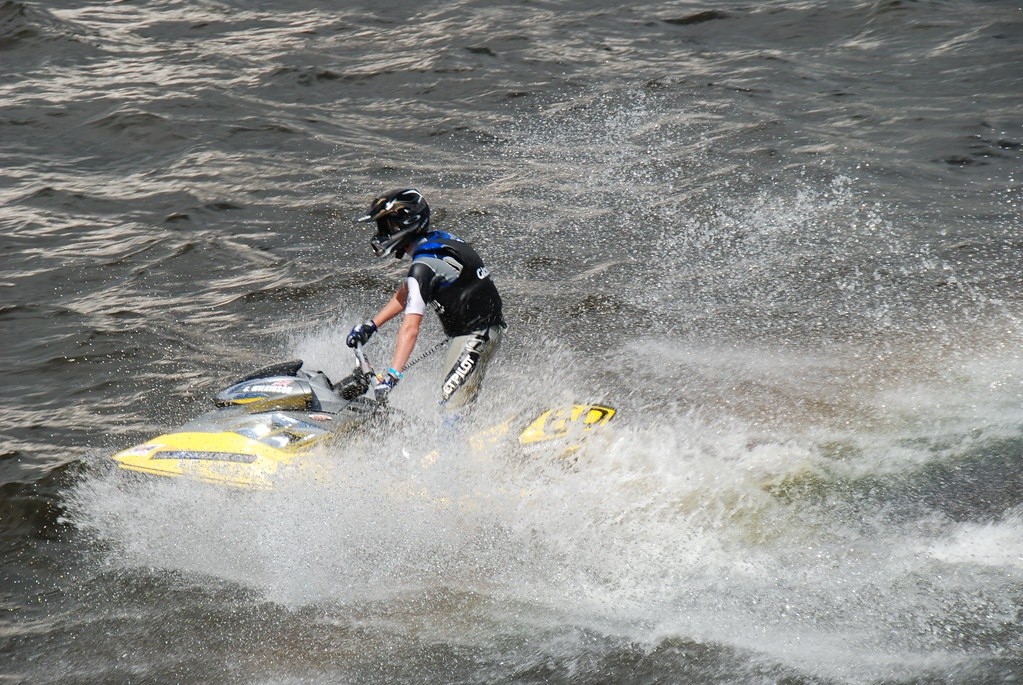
345;320;378;348
374;373;399;403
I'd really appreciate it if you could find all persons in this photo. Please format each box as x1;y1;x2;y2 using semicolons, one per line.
346;186;508;432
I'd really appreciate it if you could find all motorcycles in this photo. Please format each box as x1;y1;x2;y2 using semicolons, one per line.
111;335;617;517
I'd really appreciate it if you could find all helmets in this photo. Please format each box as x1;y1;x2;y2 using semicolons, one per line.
356;186;431;259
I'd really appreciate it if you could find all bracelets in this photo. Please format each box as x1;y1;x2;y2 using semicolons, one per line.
389;368;403;380
